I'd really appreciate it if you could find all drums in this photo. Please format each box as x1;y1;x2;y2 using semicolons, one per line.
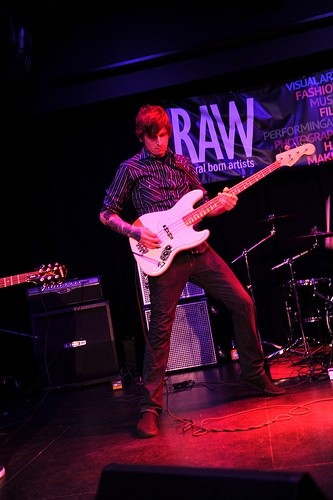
282;278;333;320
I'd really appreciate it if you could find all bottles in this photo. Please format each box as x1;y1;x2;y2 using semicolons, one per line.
230;339;239;362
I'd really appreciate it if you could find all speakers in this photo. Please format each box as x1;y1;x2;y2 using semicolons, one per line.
30;301;121;393
97;462;329;500
143;301;217;375
137;264;206;305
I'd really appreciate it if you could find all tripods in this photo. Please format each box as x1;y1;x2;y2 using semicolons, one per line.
232;231;282;350
270;249;327;357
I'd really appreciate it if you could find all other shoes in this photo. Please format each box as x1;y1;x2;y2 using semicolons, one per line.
241;372;287;396
137;410;160;437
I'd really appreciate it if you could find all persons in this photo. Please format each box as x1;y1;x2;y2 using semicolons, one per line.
101;106;285;438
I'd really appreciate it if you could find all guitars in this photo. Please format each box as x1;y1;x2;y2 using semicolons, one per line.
128;142;316;275
257;215;294;222
0;262;68;289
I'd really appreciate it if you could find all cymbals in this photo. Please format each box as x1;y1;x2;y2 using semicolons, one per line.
299;232;333;242
